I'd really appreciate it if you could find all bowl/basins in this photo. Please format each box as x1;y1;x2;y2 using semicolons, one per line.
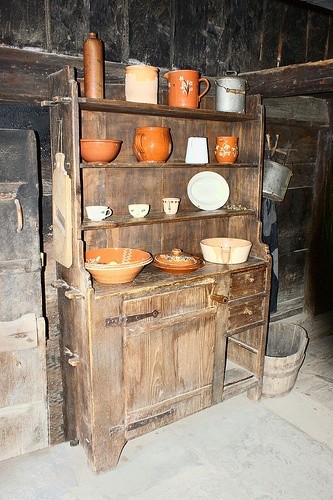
151;248;206;275
199;238;252;265
128;204;149;218
84;248;153;284
132;127;172;164
79;138;123;164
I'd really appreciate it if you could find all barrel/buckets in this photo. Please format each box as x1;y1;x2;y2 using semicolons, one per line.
213;76;246;114
262;152;293;202
261;322;309;398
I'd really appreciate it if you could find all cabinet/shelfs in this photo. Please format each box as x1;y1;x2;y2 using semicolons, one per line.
42;67;271;472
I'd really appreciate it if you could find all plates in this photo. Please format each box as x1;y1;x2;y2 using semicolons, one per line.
187;171;230;211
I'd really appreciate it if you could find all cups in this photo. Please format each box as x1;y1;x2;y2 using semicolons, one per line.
124;65;159;104
185;137;208;164
86;205;113;222
161;197;180;214
163;69;209;109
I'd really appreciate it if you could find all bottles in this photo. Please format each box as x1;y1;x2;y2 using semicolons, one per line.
214;136;239;165
83;32;104;99
